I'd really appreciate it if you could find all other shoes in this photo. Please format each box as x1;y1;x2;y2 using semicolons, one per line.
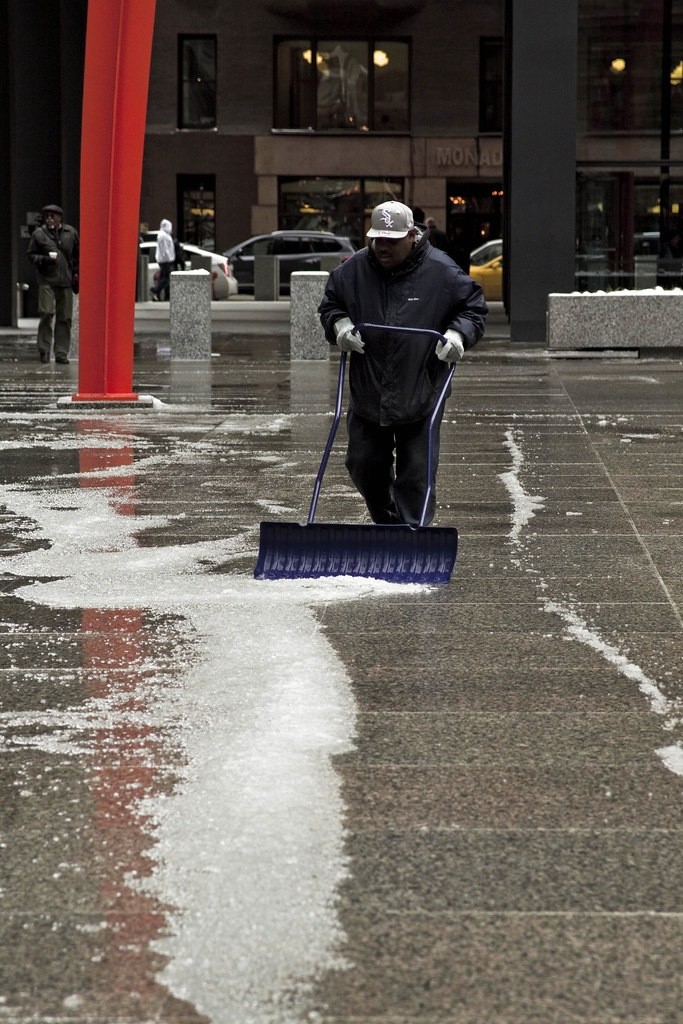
55;355;69;364
149;287;159;301
40;349;50;364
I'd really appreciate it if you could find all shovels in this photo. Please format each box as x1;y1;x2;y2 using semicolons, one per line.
254;322;458;584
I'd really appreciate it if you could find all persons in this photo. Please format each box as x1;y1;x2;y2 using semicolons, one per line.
27;205;80;364
425;217;448;255
317;201;489;528
150;219;186;301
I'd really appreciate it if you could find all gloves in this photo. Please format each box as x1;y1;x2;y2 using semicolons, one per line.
435;326;465;362
333;318;366;354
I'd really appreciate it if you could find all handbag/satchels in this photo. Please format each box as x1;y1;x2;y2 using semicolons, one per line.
71;270;80;296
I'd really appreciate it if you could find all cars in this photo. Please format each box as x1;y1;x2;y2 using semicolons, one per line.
469;240;504;303
138;241;239;301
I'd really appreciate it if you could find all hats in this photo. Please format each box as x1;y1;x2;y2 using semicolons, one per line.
366;200;413;238
41;204;62;215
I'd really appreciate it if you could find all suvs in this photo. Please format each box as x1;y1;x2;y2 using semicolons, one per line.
225;230;354;293
